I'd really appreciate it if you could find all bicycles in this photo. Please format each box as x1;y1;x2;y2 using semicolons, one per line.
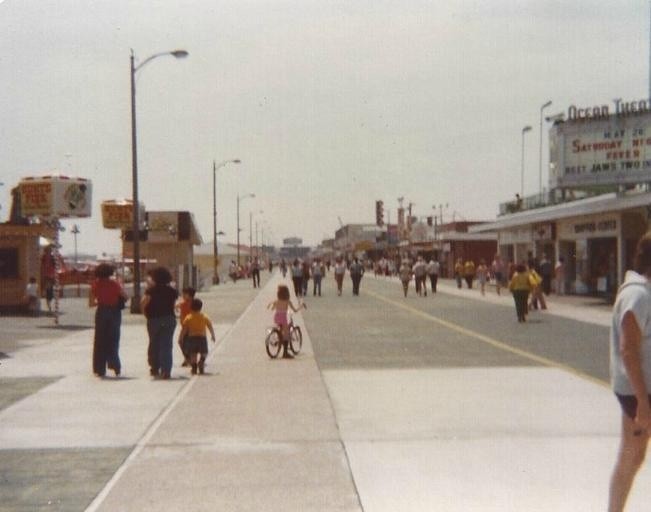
263;302;313;360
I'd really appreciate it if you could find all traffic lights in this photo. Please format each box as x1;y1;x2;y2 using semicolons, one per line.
375;200;385;227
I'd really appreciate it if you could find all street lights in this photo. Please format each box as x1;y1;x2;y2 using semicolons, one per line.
522;125;533;214
234;191;274;272
212;157;242;285
539;98;554;209
127;49;189;317
432;203;451;225
71;223;81;263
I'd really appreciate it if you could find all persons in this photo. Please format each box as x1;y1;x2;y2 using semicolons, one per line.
23;276;41;317
43;279;56;314
174;286;195;366
291;254;552;322
88;263;129;377
607;231;651;512
555;257;566;295
270;284;304;359
140;265;178;379
229;255;287;288
178;297;215;374
